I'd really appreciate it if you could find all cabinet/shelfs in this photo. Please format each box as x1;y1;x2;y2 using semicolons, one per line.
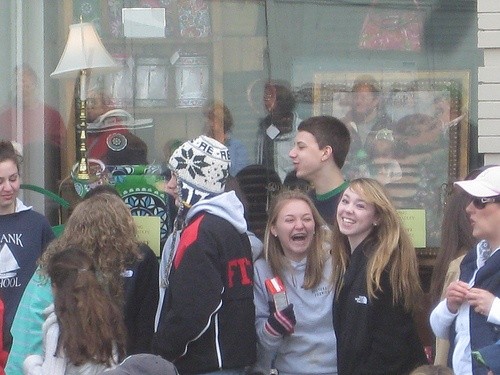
61;0;224;225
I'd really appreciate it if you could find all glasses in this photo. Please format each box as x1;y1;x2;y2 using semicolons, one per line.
473;197;500;209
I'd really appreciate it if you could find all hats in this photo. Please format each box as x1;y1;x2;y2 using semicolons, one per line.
453;166;500;198
160;134;232;287
96;354;179;375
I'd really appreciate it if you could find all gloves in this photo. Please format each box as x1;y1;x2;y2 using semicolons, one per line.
265;301;296;336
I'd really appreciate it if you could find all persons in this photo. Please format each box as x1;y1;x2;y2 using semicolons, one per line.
145;135;257;375
339;76;393;163
82;185;160;355
23;248;127;375
252;190;336;375
0;63;66;148
0;193;144;375
254;83;302;184
429;165;500;375
330;178;429;375
354;137;402;183
0;140;55;348
87;87;128;165
202;101;253;177
288;116;351;225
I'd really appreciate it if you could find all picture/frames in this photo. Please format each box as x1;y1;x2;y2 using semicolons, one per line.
312;71;470;270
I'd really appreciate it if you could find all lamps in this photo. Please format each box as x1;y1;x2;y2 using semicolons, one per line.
50;14;118;179
